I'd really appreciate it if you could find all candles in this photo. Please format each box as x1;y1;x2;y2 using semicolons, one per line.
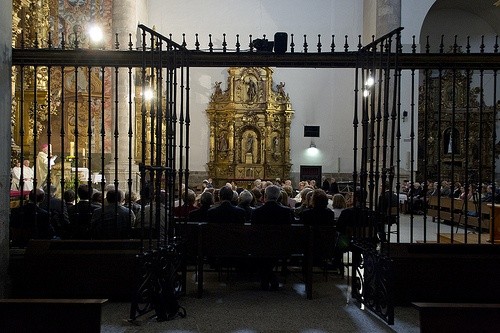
82;147;85;156
70;142;75;156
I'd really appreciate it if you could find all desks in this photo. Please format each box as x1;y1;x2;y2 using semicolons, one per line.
0;299;108;333
337;181;353;192
412;302;500;333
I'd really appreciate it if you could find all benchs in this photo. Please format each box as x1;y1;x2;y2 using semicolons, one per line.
10;206;397;307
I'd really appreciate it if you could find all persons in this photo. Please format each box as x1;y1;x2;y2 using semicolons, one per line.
11;162;21;191
22;160;34;191
337;189;379;279
37;144;55;188
10;177;348;289
376;182;399;240
402;180;500;232
